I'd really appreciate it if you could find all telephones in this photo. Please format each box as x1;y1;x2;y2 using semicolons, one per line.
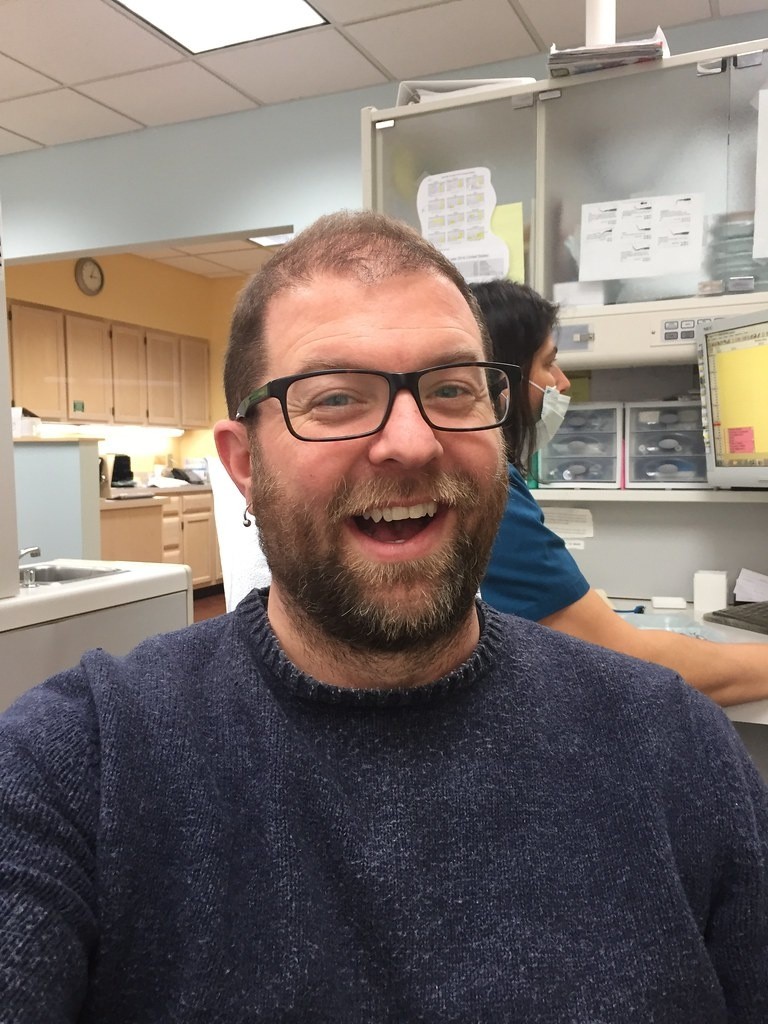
173;467;201;482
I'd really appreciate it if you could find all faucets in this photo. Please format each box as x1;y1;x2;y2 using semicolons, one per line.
18;547;41;558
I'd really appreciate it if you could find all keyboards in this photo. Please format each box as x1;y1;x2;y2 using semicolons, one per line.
701;600;768;634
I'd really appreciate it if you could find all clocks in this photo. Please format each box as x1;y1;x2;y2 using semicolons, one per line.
75;256;105;296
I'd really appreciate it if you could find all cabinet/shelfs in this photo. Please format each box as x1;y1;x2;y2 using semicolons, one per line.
100;492;224;590
5;297;212;430
359;36;768;503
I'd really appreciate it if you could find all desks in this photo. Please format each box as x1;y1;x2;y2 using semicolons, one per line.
609;597;768;726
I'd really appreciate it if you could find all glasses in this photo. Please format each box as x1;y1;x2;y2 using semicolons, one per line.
236;361;524;442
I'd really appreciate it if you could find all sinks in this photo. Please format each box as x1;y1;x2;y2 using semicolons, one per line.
19;564;132;585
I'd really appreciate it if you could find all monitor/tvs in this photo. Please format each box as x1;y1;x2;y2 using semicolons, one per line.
693;308;768;486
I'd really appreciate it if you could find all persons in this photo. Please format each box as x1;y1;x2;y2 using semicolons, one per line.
0;209;768;1024
464;279;768;708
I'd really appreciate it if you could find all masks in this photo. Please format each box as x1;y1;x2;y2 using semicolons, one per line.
501;379;572;465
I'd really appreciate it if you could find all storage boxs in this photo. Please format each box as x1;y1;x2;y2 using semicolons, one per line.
623;401;714;489
552;280;623;307
538;400;624;489
